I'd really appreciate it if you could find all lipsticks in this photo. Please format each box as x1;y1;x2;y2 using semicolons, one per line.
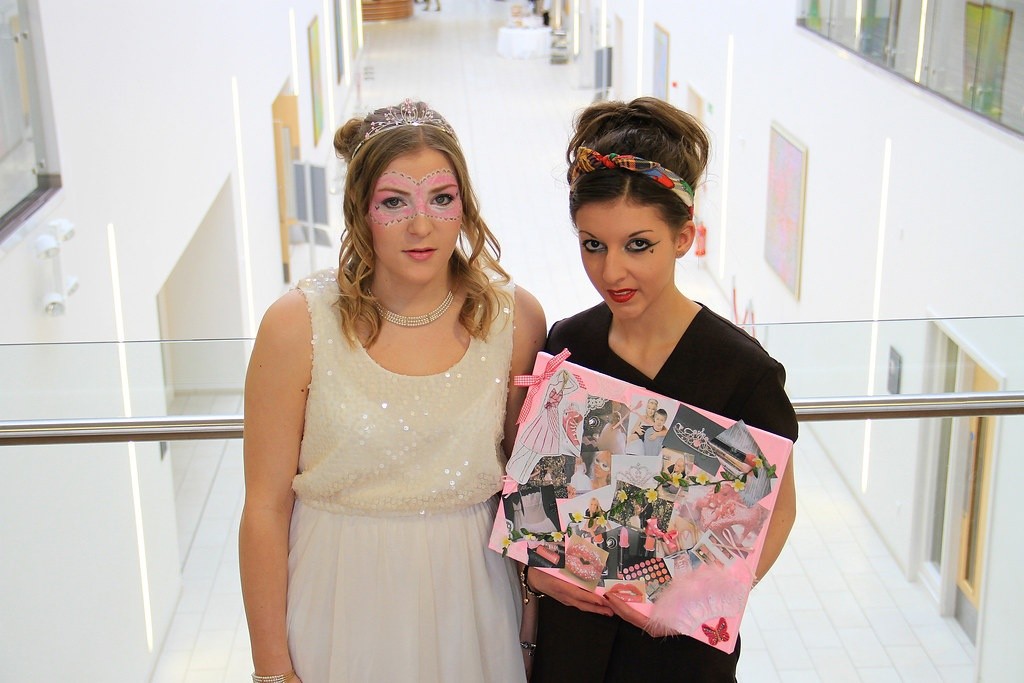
593;534;608;573
642;535;655;564
618;526;630;575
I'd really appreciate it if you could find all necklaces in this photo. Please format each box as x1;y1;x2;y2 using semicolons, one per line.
365;284;455;327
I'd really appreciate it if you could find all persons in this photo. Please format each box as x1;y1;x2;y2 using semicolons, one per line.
666;456;690;496
597;399;669;455
237;96;548;683
517;96;799;683
544;451;612;493
584;497;612;534
630;496;654;528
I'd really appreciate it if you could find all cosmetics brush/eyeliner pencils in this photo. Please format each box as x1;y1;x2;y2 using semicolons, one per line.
708;436;757;483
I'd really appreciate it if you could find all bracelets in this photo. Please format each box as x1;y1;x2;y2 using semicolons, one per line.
520;641;537;657
520;564;546;605
251;669;295;683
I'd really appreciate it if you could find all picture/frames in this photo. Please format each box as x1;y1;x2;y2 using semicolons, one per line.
761;123;808;297
